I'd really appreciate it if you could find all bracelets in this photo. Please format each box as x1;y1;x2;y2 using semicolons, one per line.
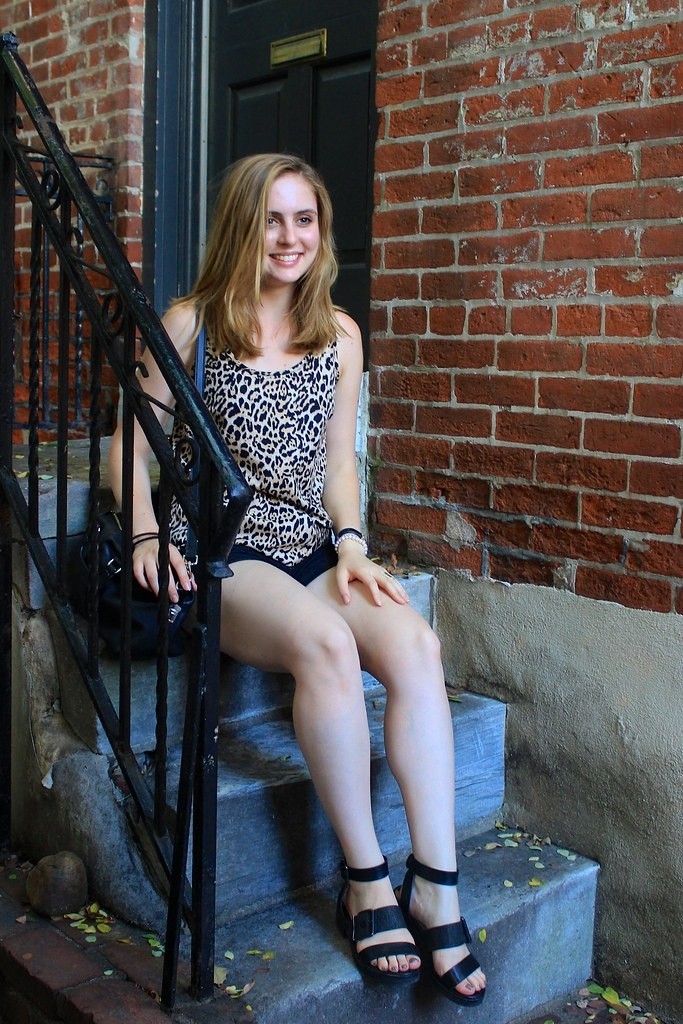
335;533;368;556
132;532;158;540
133;536;158;546
335;528;367;544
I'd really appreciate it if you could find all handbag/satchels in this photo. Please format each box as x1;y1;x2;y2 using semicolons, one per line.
68;510;195;661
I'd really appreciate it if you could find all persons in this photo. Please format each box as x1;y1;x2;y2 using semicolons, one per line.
108;152;488;1007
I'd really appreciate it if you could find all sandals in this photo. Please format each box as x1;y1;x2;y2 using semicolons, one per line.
394;853;487;1007
335;856;422;984
